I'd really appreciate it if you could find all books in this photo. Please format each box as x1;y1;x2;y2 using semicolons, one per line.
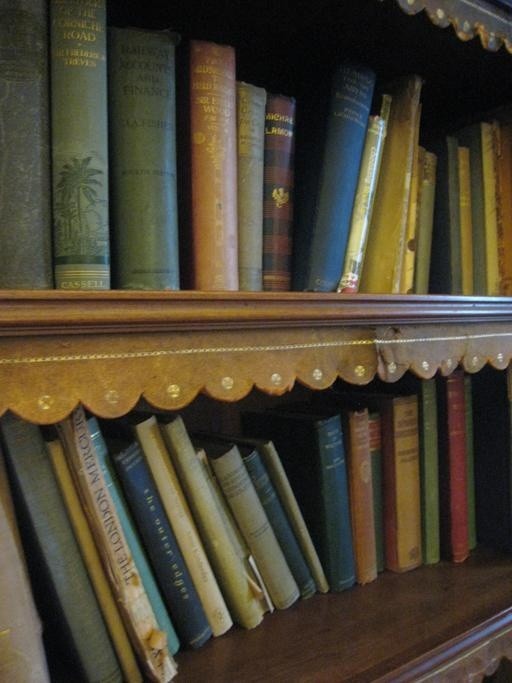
239;377;476;595
0;369;328;682
1;0;512;295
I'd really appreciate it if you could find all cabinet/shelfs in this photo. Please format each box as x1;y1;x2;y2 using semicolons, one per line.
1;0;512;683
